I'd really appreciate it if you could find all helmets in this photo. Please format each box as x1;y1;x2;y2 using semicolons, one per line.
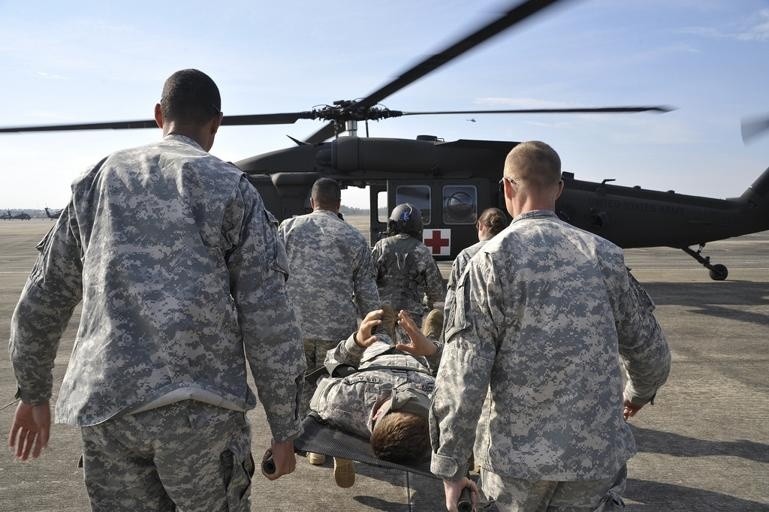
387;203;423;241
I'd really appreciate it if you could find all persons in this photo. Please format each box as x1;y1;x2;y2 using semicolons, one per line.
306;299;443;464
8;68;308;511
370;202;446;330
441;208;508;342
428;141;671;511
276;176;382;488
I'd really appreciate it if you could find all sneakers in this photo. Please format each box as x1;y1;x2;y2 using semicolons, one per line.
333;456;356;488
379;301;395;332
306;452;325;465
424;309;444;339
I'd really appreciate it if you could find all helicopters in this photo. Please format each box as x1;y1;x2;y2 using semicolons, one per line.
0;208;31;221
0;1;768;282
45;207;64;221
